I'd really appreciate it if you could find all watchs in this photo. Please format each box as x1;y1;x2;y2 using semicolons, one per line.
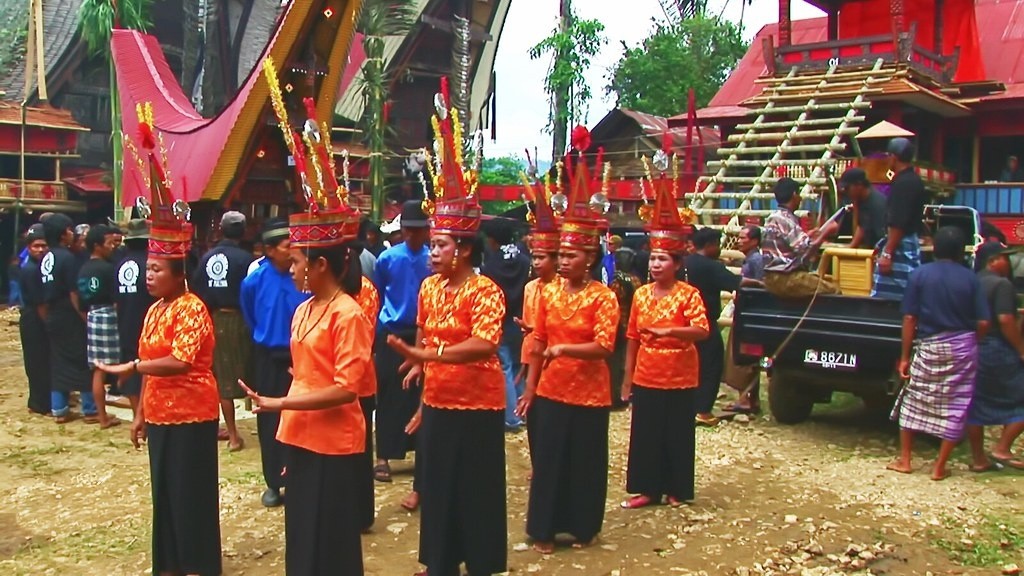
880;252;893;259
134;357;141;371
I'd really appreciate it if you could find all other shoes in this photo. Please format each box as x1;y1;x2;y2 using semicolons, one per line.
665;494;681;506
692;411;719;426
262;485;280;507
621;491;662;509
216;426;228;439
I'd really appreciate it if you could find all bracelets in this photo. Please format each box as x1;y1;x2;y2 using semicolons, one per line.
436;342;445;362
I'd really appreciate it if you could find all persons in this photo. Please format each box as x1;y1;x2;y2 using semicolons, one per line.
885;224;992;480
396;209;443;511
869;137;932;301
89;220;225;576
295;207;377;534
963;242;1024;473
838;167;887;253
763;176;845;299
237;204;375;576
387;211;509;576
15;210;764;503
509;225;563;417
617;224;710;509
512;221;622;554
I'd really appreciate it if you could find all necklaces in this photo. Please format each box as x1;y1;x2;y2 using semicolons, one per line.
140;289;186;341
431;270;471;321
295;286;341;344
555;277;595;320
533;273;560;316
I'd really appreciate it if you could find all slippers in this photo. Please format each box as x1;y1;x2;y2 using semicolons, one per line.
54;411;83;422
100;418;121;429
723;401;762;415
27;405;54;418
375;461;393;482
987;450;1024;472
229;435;244;452
83;412;116;424
401;491;419;511
968;458;991;473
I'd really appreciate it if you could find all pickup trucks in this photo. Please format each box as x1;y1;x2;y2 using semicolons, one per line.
729;202;1024;434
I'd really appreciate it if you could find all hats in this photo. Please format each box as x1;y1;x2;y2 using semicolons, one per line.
218;211;247;228
122;217;151;243
399;199;430;228
380;215;401;234
975;240;1019;261
260;221;289;242
839;168;865;188
27;225;46;243
105;55;696;261
